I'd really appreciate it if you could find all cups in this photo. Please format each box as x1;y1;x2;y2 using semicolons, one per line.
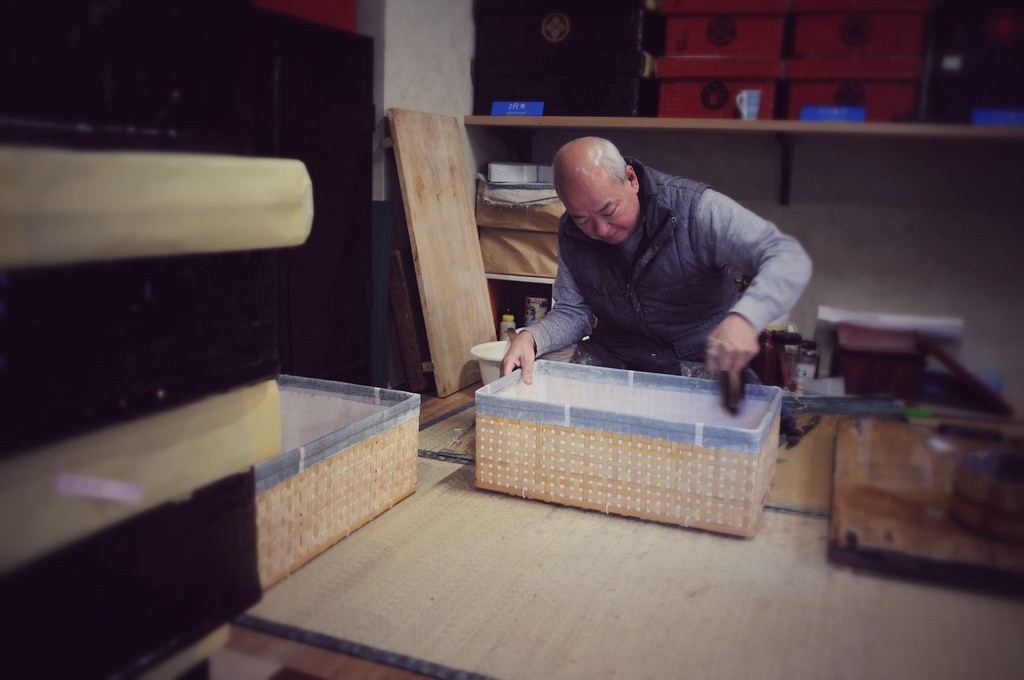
737;89;760;120
524;296;548;327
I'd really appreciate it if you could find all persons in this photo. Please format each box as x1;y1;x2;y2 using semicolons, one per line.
500;137;814;386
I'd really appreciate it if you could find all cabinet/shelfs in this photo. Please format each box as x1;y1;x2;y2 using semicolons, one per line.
465;0;1024;314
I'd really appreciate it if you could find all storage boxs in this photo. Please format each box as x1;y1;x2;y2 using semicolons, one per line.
810;299;1016;415
467;0;1024;129
469;355;784;543
0;369;424;680
475;156;569;283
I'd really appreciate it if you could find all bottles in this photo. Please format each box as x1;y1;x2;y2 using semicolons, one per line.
933;56;973;125
755;316;819;393
500;315;516;342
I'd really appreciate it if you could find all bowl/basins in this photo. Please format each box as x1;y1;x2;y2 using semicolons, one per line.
470;341;510;386
